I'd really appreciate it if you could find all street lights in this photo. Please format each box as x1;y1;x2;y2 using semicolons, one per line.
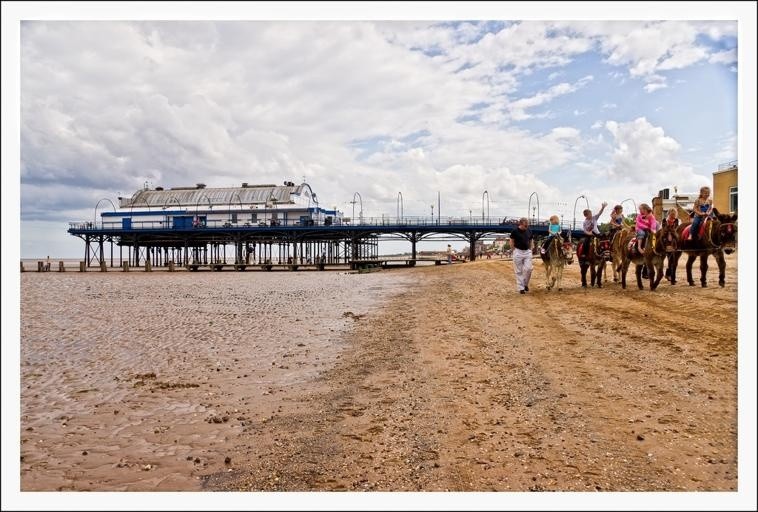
532;206;536;226
333;204;337;225
469;208;472;224
560;214;564;228
430;203;435;225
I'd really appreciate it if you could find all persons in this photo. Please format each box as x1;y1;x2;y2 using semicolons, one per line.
661;206;682;232
541;215;563;255
686;186;713;241
580;201;608;262
510;217;534;295
634;203;661;254
607;205;633;245
452;248;511;261
248;244;255;262
446;244;452;264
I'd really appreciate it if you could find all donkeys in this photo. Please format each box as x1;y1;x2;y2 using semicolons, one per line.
677;207;737;287
617;218;679;291
603;226;636;283
540;230;574;291
577;229;614;287
